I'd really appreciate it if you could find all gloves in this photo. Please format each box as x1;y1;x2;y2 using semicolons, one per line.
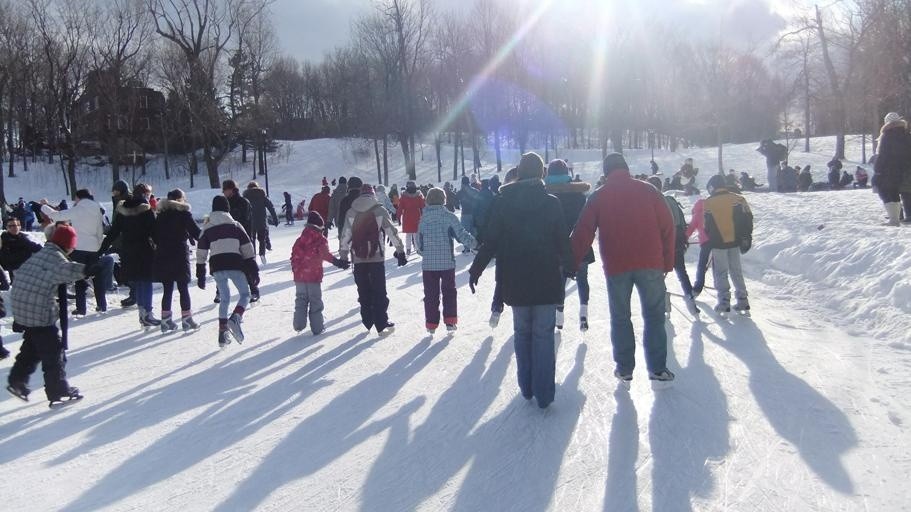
252;271;260;286
198;275;205;289
740;234;752;254
332;257;350;270
469;274;478;294
398;253;405;266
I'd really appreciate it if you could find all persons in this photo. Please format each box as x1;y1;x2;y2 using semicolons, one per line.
871;112;908;226
885;133;911;222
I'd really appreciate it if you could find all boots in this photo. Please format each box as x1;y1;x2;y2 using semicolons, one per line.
733;298;750;310
137;304;201;331
881;202;901;226
219;314;244;344
121;290;136;306
715;299;730;312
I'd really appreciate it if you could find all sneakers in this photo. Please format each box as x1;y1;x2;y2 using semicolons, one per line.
250;289;259;303
615;369;632;380
96;306;106;311
649;368;674;381
72;308;86;315
9;374;31;395
214;288;221;303
580;317;588;332
48;387;79;400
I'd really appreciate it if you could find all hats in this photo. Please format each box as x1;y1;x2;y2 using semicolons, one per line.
212;196;230;212
112;180;127;194
603;154;629;178
339;177;385;195
52;223;77;249
884;112;900;124
425;188;447;205
308;211;324;227
504;152;568;184
223;180;235;189
